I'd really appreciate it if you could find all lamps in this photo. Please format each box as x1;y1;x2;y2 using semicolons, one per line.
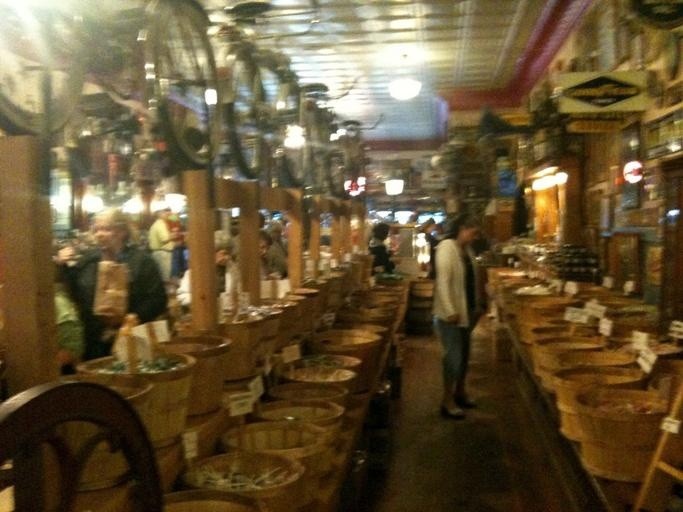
381;176;408;195
388;76;422;101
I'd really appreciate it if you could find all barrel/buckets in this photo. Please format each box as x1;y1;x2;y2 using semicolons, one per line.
58;253;410;512
411;283;436;334
485;262;679;483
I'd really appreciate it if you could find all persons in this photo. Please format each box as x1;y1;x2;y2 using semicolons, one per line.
174;210;290;322
433;212;487;421
368;221;399;277
49;205;191;374
423;232;439;281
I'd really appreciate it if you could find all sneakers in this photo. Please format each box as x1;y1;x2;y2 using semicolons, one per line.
441;393;477;419
